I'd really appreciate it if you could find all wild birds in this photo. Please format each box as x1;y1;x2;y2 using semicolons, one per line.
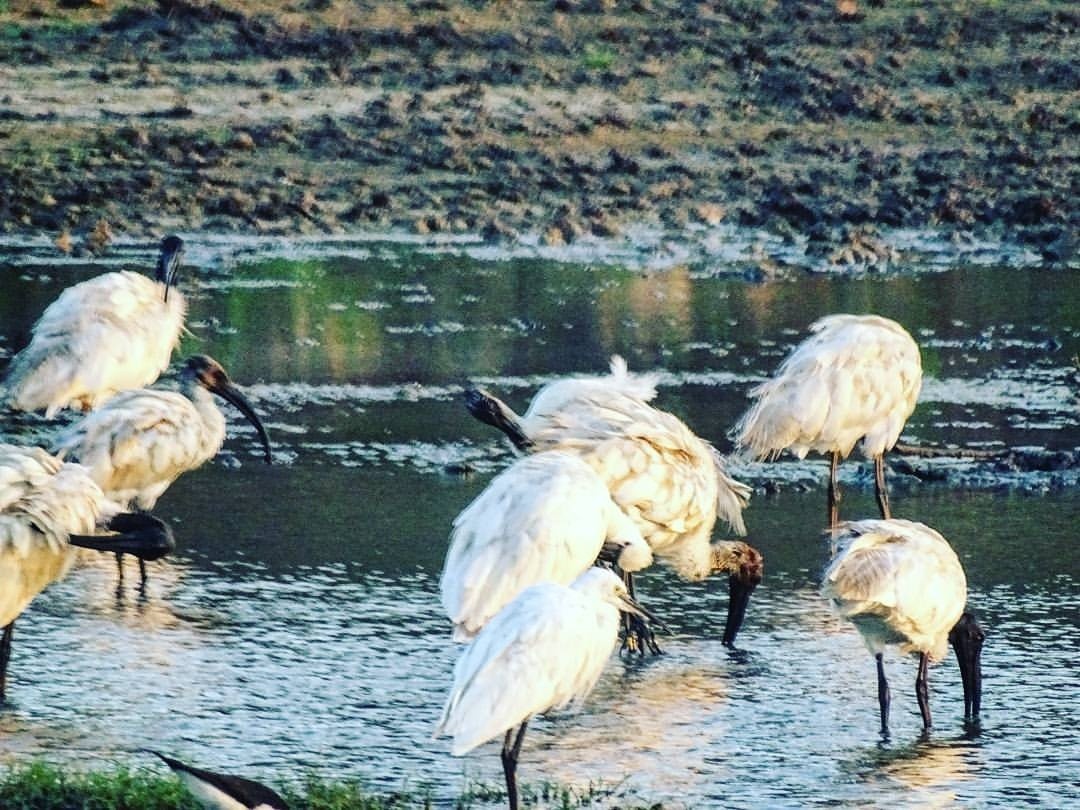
432;568;675;810
146;748;289;810
465;354;764;645
0;442;176;702
820;516;986;734
33;353;271;586
0;234;190;431
439;452;653;650
726;314;923;525
